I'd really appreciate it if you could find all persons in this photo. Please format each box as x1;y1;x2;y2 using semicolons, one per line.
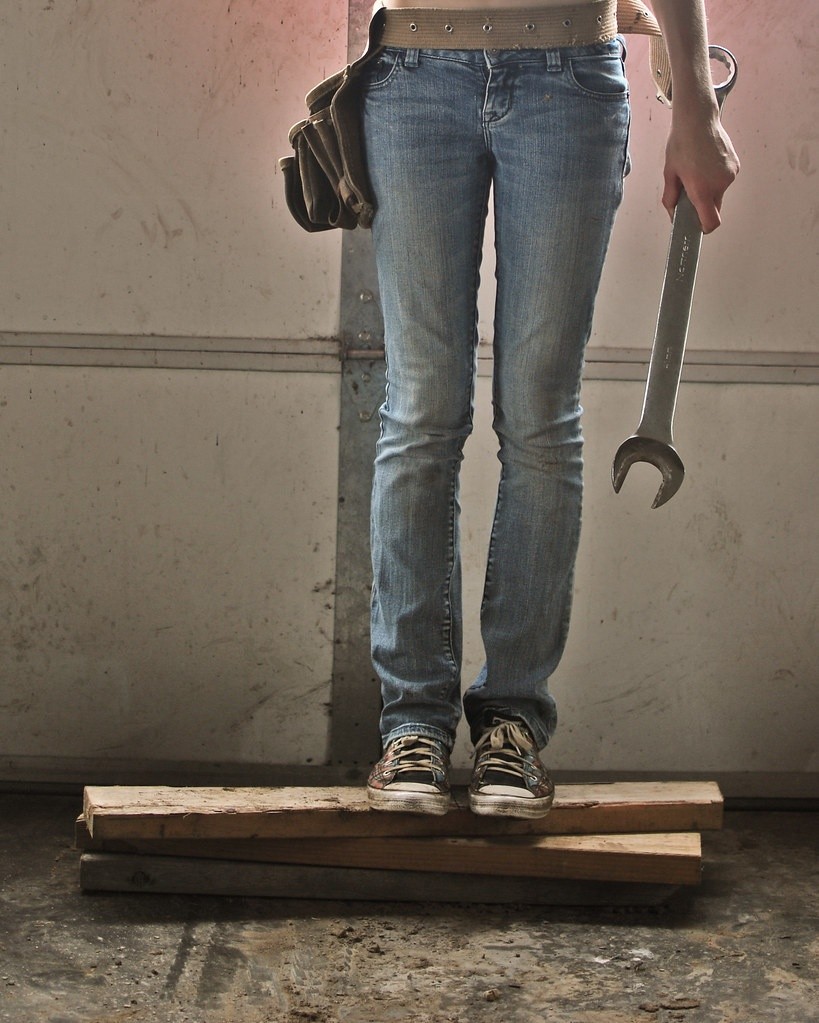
355;0;745;823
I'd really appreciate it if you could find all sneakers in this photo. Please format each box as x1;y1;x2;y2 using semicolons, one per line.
367;736;453;817
469;708;555;818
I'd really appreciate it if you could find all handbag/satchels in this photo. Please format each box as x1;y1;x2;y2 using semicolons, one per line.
278;0;388;233
